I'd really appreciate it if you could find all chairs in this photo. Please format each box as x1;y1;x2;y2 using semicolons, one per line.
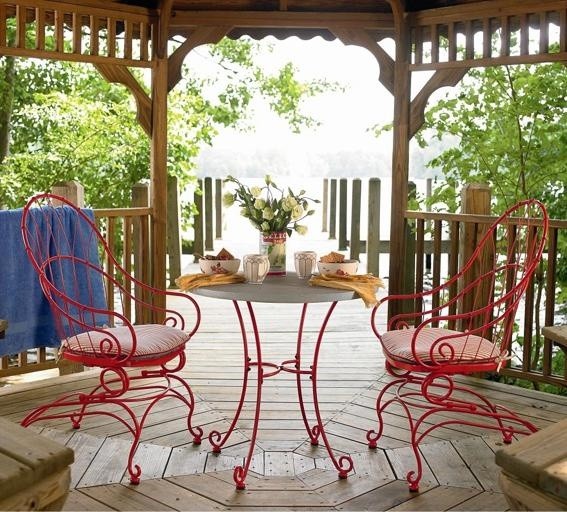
21;193;203;485
365;199;549;493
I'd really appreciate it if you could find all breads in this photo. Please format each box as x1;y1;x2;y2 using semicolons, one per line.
320;252;345;263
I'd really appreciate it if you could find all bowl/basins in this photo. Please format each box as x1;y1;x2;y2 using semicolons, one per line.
318;259;360;276
198;256;241;276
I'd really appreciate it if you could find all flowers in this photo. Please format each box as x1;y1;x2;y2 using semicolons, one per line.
220;173;322;237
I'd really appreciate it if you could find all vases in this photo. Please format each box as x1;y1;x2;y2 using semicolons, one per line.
257;232;286;281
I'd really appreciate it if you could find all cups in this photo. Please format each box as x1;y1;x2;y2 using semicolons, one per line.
243;253;271;284
294;250;318;280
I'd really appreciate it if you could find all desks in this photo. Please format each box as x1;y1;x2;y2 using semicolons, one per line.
175;270;377;491
